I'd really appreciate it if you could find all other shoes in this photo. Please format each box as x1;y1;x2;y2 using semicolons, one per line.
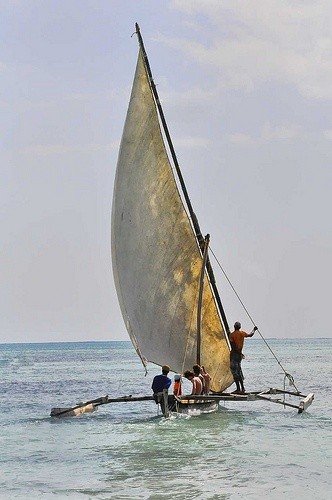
230;388;246;394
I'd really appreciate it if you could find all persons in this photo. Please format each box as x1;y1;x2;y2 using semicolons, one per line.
174;363;212;395
151;364;171;394
229;321;258;394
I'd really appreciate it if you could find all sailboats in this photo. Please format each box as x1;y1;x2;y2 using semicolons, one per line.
48;20;317;417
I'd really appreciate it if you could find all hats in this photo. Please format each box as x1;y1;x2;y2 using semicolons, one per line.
234;322;241;328
162;366;170;371
